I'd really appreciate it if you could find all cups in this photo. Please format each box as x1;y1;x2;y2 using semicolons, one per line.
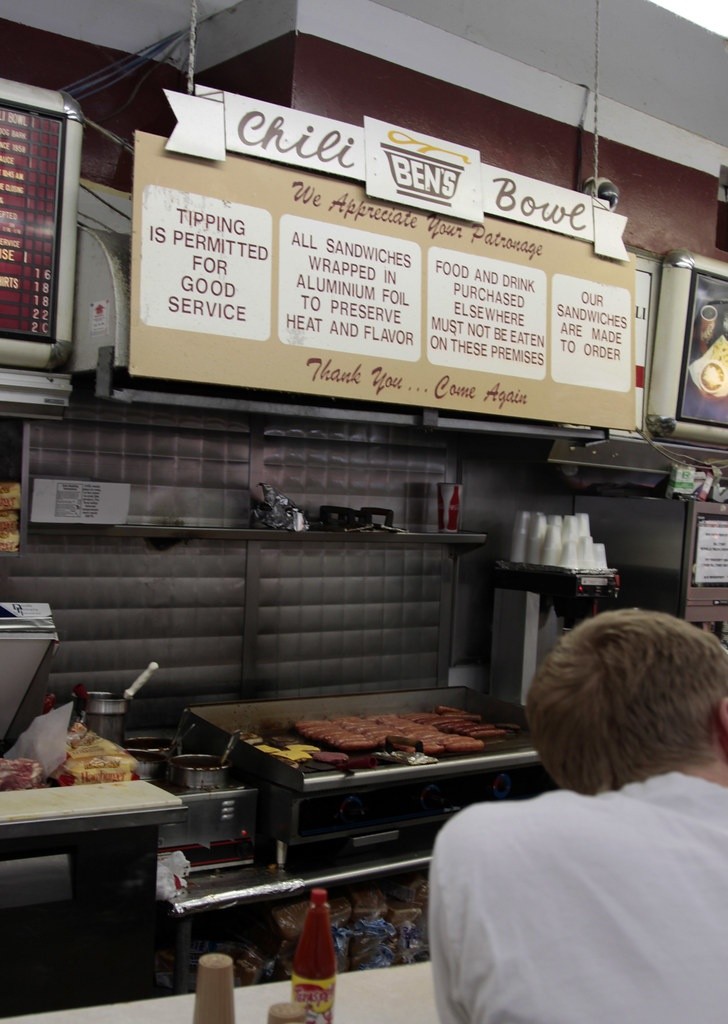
436;483;465;532
510;510;608;568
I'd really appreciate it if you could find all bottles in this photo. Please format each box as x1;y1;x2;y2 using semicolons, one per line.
292;888;337;1024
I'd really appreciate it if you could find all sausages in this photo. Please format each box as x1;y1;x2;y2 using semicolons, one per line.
293;705;521;756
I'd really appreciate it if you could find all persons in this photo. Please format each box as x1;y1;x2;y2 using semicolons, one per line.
429;608;728;1024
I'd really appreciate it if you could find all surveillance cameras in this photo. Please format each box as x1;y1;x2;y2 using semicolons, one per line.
585;177;619;213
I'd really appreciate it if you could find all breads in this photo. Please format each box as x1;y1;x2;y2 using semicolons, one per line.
157;876;429;989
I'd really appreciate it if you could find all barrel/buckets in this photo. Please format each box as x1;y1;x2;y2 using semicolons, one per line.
71;692;128;746
